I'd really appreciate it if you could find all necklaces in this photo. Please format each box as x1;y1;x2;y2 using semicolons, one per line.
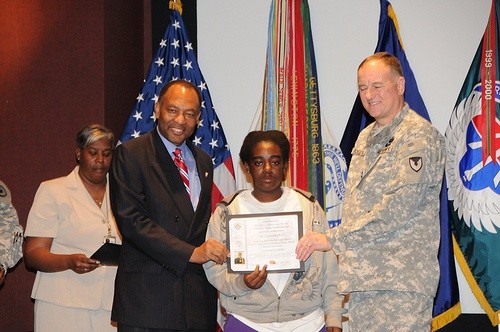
81;179;107;205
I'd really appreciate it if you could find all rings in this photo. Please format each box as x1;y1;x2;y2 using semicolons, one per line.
84;268;87;272
306;244;311;248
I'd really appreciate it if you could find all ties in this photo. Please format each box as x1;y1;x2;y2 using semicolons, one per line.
172;148;191;200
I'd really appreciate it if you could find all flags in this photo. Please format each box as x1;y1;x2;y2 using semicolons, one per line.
443;0;500;326
237;0;351;228
115;10;238;332
340;0;462;332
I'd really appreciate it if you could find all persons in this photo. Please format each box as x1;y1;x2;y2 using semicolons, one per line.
109;80;230;332
295;52;446;332
202;129;344;332
0;181;25;287
22;123;122;332
